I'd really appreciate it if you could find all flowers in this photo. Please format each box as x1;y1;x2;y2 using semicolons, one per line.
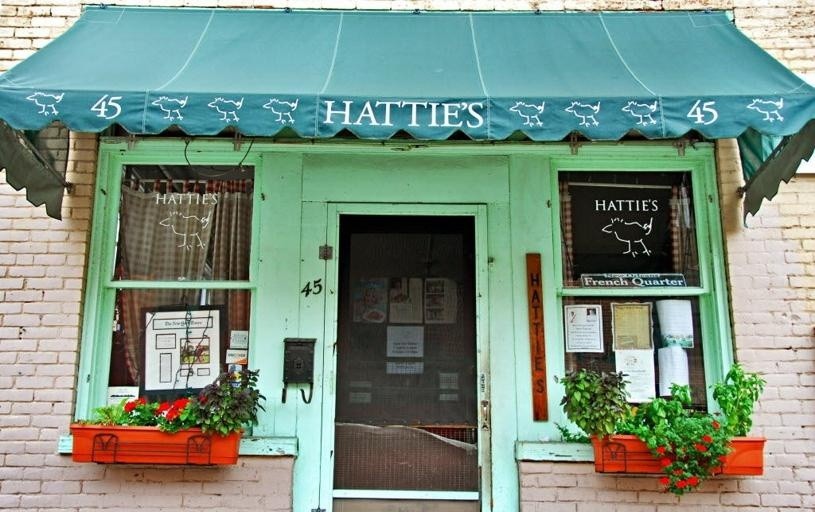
89;368;267;436
557;364;766;496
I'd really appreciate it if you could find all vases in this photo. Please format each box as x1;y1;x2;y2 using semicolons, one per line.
589;434;766;478
71;420;244;466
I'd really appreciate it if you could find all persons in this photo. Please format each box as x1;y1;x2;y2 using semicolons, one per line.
389;280;403;303
